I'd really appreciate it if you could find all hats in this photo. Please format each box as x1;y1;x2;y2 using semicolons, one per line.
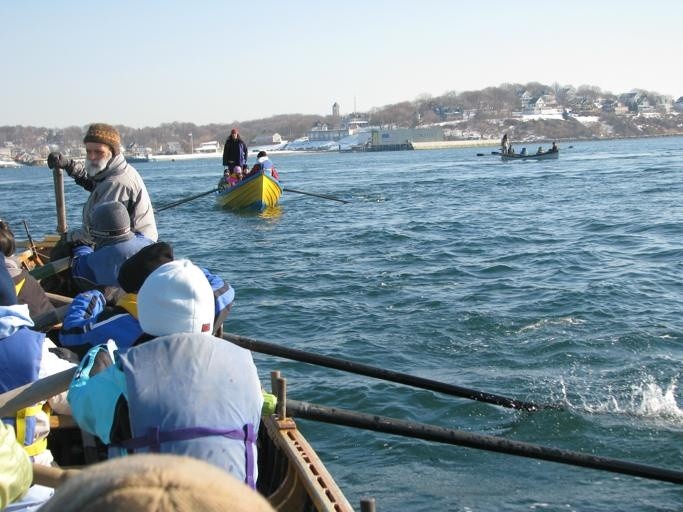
80;122;123;157
86;200;131;240
114;242;178;300
134;257;215;338
34;449;278;512
231;128;238;135
223;151;267;176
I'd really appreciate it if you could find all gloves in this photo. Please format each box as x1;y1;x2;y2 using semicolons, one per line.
45;152;69;171
93;283;119;305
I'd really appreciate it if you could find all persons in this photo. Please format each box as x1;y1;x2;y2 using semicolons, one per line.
1;198;279;511
248;151;279;180
48;123;159;279
223;129;248;174
502;134;557;155
218;165;249;193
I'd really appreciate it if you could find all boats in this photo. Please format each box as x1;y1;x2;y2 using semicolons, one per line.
124;156;150;163
498;148;560;163
11;231;355;510
215;171;284;214
